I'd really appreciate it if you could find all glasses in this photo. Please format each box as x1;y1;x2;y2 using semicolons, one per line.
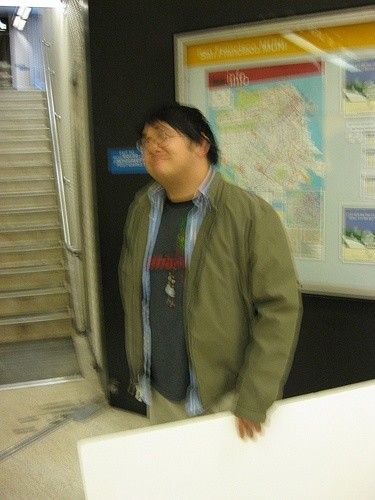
135;128;192;153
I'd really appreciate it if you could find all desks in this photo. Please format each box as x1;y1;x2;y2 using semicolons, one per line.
76;381;375;500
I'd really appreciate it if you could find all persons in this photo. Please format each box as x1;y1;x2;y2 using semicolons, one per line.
116;102;303;439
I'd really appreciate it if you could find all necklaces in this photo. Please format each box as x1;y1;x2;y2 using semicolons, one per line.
161;187;198;298
